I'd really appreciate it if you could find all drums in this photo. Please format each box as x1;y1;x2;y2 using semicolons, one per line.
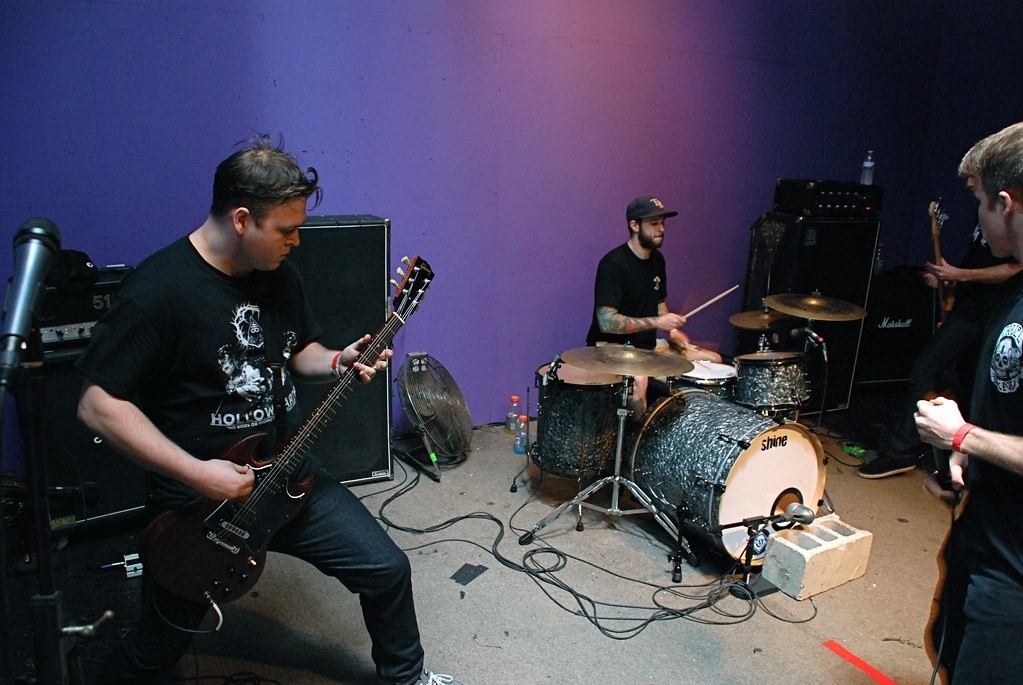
534;361;635;481
665;358;738;402
629;386;829;567
735;350;813;410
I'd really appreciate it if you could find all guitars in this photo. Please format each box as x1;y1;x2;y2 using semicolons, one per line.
139;253;436;606
928;198;959;328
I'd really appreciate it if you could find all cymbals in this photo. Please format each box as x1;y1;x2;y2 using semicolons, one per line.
559;344;694;379
761;290;869;323
728;309;805;331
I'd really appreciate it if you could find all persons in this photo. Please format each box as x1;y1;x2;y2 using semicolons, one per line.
912;120;1023;685
586;197;722;411
858;156;1023;499
76;128;461;684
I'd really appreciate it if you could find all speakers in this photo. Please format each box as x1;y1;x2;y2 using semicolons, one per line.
733;213;882;419
11;355;150;538
284;213;393;488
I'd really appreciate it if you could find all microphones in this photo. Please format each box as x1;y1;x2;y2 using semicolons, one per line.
785;503;814;525
791;327;823;342
0;217;62;387
934;446;955;508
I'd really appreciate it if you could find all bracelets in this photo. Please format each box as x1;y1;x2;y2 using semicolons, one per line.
330;352;342;379
952;423;976;452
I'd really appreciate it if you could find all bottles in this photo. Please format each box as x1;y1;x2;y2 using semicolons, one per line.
505;395;530;454
875;242;885;274
859;150;874;185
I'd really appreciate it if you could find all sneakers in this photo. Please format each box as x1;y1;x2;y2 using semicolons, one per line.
410;668;466;685
857;456;917;480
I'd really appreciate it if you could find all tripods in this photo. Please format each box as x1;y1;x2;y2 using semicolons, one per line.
518;374;702;569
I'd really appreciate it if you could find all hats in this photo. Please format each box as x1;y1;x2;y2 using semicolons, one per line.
625;196;678;220
